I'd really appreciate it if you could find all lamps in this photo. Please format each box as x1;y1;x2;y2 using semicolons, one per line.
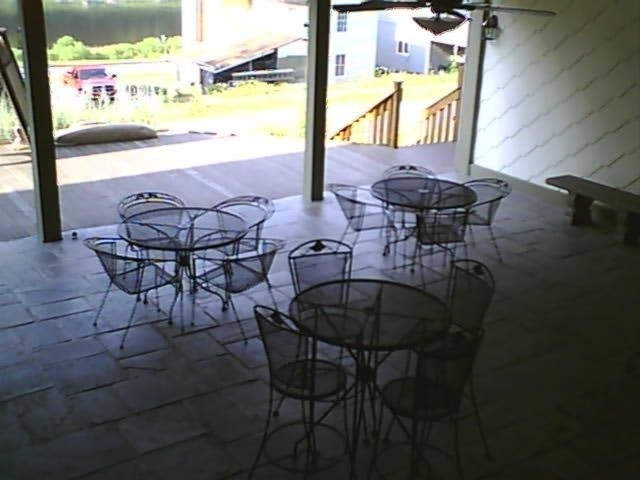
412;12;464;37
482;15;500;40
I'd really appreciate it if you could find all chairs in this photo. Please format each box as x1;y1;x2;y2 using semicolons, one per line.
83;236;180;350
384;260;496;444
192;243;279;348
247;304;353;480
383;164;441;252
367;328;492;477
442;178;512;265
274;239;378;436
116;192;192;302
409;209;469;273
329;184;397;268
193;195;272;309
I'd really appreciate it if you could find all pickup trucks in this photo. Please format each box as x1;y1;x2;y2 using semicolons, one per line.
64;64;117;101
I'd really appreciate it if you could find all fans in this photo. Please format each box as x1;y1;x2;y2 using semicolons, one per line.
332;0;558;21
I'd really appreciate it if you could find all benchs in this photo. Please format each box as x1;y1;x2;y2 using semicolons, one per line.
545;173;640;243
